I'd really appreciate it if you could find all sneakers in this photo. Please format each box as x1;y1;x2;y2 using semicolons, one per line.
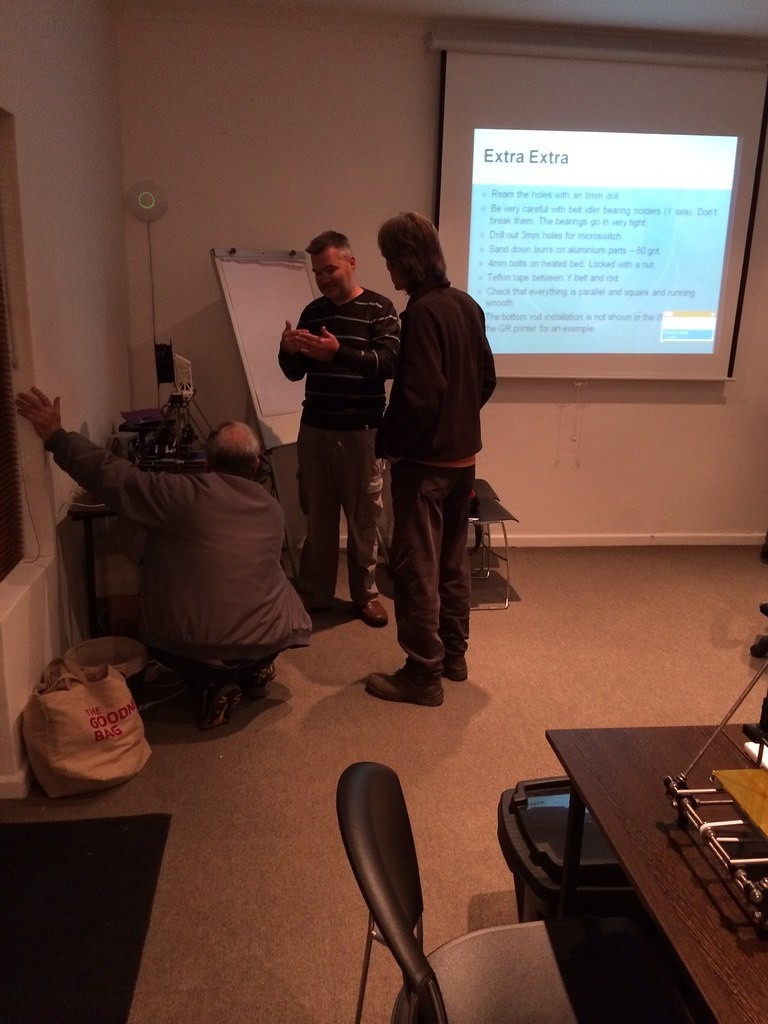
196;679;242;730
243;660;277;698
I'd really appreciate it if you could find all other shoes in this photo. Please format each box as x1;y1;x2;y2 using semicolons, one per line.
441;652;468;682
368;668;444;706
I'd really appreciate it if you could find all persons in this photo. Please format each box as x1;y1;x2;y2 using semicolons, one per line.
277;230;400;628
365;212;497;708
15;386;312;730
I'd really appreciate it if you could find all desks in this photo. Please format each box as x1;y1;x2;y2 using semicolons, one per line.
544;722;768;1024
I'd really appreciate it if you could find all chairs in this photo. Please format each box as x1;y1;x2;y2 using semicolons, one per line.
468;478;502;574
467;498;520;611
336;761;695;1024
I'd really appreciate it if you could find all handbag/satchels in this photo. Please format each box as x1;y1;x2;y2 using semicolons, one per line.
22;657;152;800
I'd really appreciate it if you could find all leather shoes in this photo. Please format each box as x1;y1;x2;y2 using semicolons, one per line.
354;597;388;627
294;588;330;613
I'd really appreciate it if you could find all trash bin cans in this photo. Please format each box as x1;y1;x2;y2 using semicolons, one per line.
497;774;667;923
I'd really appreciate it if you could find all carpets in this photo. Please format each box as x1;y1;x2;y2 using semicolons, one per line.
0;812;173;1024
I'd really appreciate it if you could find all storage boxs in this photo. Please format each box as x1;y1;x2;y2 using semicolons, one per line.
497;774;643;923
